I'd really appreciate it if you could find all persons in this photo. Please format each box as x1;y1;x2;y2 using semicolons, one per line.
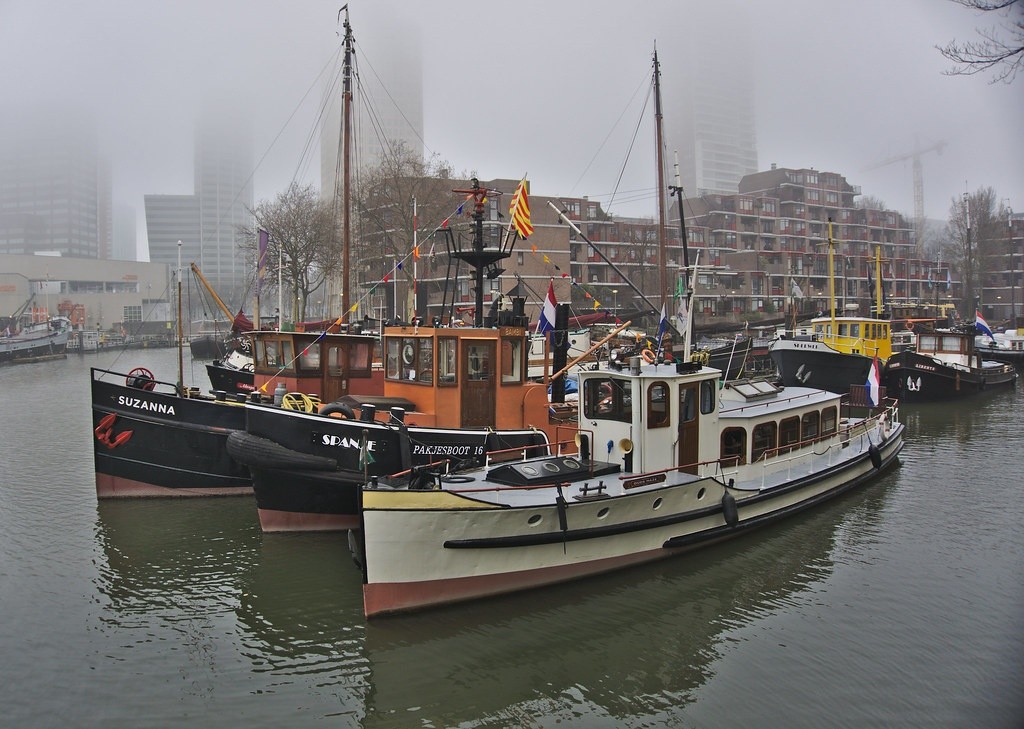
275;308;279;325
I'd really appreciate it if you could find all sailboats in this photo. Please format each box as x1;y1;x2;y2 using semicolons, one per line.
88;5;434;501
767;217;893;395
349;39;905;618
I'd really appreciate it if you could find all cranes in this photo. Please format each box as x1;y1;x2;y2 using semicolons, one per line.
878;138;941;260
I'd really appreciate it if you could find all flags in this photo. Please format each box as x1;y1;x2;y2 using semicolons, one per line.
539;282;571;351
928;270;932;288
976;309;994;340
791;278;803;299
674;276;684;300
864;356;881;406
677;301;688;336
658;302;667;354
509;177;535;240
947;270;951;291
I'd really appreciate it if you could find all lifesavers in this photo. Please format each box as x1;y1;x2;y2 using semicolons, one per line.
905;322;915;331
641;349;656;364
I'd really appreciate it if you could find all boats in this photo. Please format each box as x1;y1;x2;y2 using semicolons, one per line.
0;291;70;359
885;250;1019;402
226;176;614;534
